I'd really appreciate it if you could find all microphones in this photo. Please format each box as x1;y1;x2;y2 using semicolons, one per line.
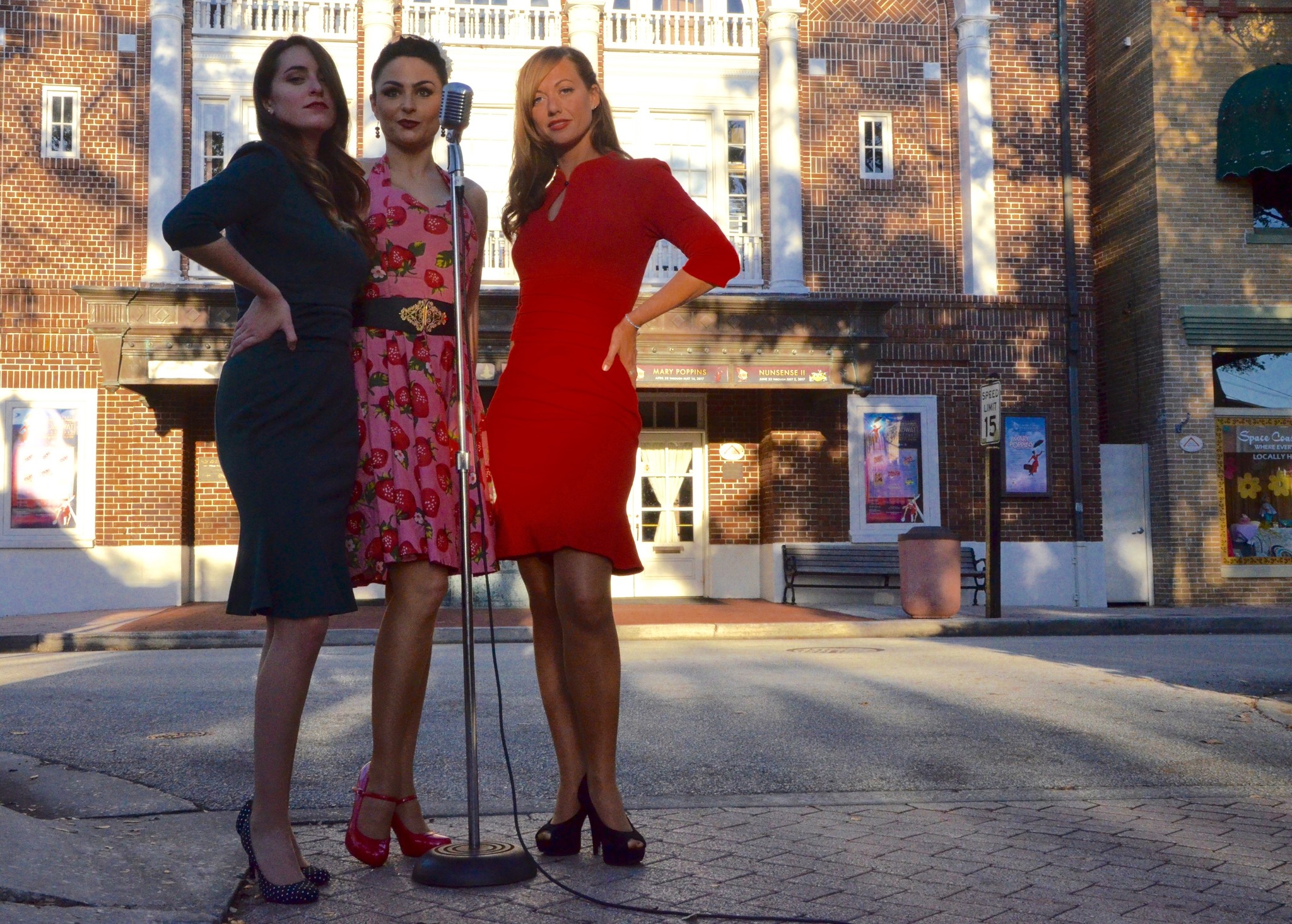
440;82;474;131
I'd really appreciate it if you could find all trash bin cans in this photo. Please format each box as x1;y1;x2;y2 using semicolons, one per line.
898;526;961;618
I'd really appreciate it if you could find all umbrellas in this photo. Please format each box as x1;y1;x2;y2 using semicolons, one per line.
1032;440;1046;453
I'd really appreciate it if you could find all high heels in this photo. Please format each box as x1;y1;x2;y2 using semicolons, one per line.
577;772;647;866
236;799;332;885
535;771;587;855
240;819;319;904
345;761;397;867
391;795;451;856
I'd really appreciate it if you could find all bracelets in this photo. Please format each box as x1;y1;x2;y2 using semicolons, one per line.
625;314;641;329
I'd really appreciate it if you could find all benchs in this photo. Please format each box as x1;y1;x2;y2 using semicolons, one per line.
781;543;986;601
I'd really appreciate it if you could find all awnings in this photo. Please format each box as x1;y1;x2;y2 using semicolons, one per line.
1215;63;1292;180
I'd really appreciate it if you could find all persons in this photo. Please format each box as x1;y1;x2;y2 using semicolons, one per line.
1259;496;1277;529
162;35;383;904
474;45;742;871
1028;450;1043;476
901;492;922;523
344;43;504;873
